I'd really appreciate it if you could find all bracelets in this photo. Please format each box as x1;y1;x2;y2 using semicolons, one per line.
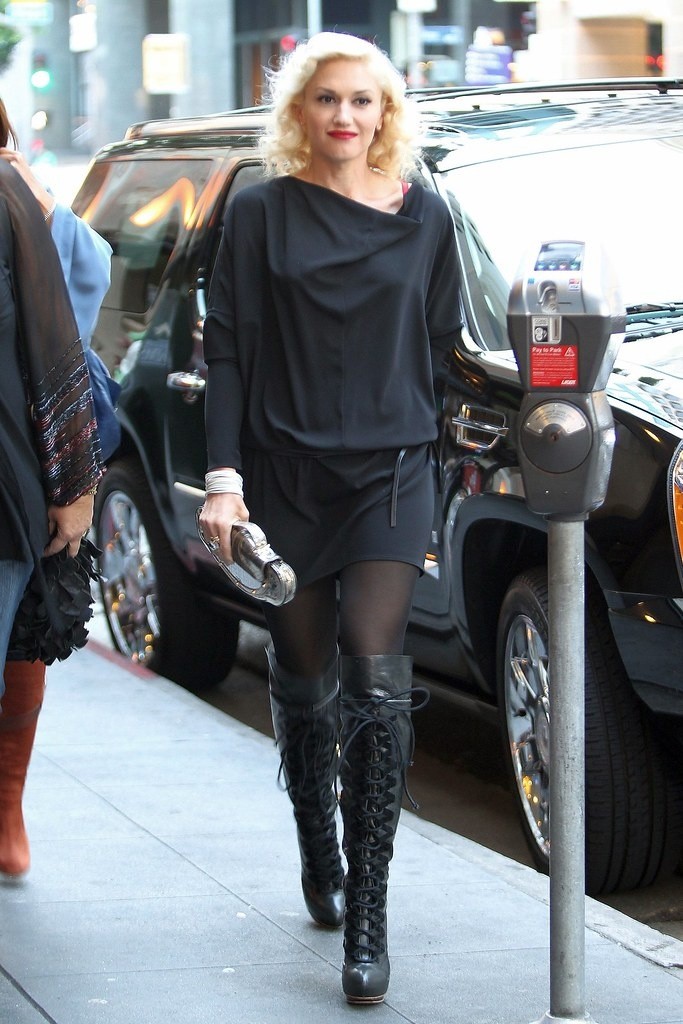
84;487;97;496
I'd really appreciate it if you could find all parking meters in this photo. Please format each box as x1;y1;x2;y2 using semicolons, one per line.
502;233;627;1024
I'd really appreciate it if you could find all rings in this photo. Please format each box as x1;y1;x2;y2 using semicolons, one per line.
210;535;219;549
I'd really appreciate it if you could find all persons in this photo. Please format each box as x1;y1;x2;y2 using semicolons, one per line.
199;30;465;1005
0;98;114;878
465;26;513;87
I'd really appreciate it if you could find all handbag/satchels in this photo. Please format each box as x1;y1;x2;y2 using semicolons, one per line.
83;349;124;463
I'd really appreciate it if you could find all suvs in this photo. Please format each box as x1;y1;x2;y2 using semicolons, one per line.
61;73;682;901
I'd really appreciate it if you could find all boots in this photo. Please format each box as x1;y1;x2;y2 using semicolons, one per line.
264;641;346;929
337;655;430;1005
0;658;46;875
